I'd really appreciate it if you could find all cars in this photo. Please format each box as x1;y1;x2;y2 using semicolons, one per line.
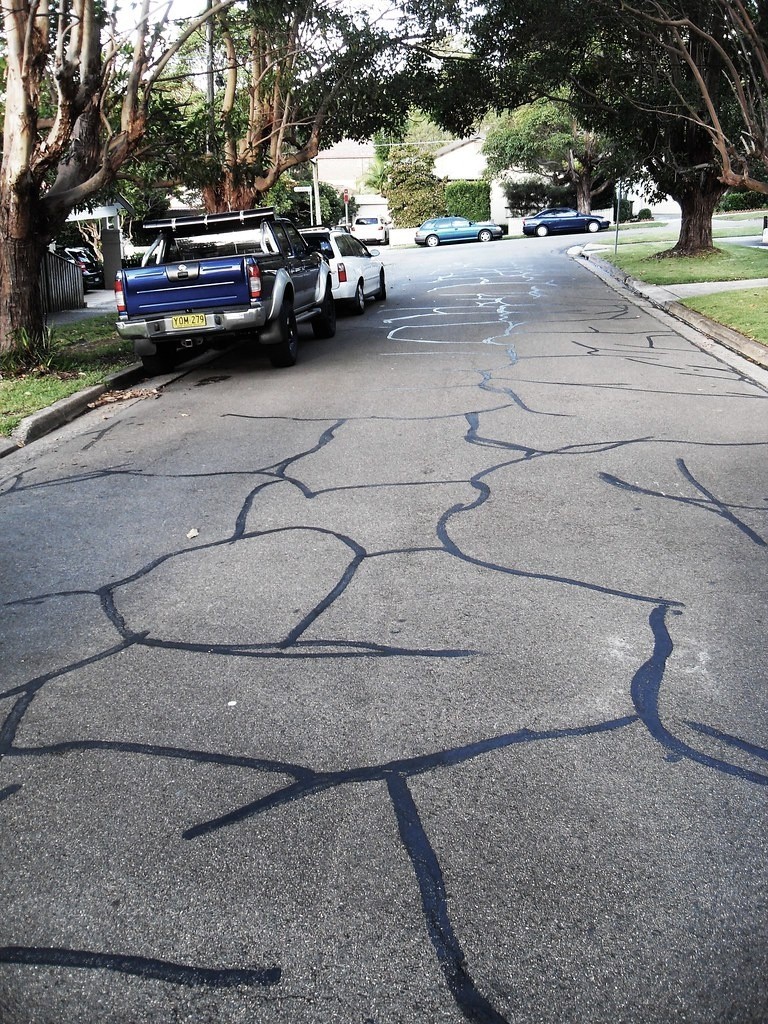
414;216;503;247
277;226;387;315
350;217;389;245
48;237;104;294
523;207;610;237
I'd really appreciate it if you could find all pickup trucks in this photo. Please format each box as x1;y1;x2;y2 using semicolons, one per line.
113;207;337;377
